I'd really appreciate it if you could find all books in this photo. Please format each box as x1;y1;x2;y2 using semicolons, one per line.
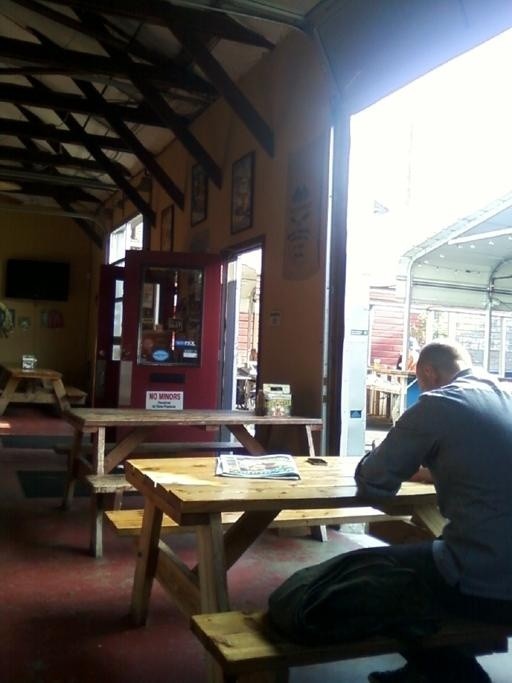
215;453;302;481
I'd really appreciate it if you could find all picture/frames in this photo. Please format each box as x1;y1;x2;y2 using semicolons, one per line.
160;205;174;252
230;151;255;235
190;163;208;227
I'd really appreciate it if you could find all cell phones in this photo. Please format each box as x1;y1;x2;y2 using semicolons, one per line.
307;458;328;466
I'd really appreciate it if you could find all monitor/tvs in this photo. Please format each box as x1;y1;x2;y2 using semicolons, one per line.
4;257;71;301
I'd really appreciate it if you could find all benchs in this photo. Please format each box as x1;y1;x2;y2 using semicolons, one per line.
190;611;401;683
85;469;419;535
61;385;88;421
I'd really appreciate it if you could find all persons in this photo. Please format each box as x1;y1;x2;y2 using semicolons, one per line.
354;336;512;683
397;337;420;369
249;349;257;360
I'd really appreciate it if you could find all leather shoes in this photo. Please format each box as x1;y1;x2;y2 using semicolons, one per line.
368;664;424;682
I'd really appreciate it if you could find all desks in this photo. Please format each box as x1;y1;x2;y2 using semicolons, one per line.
124;456;440;629
60;408;329;557
0;363;71;420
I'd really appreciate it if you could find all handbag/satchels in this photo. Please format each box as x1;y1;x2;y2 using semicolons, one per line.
267;546;428;645
